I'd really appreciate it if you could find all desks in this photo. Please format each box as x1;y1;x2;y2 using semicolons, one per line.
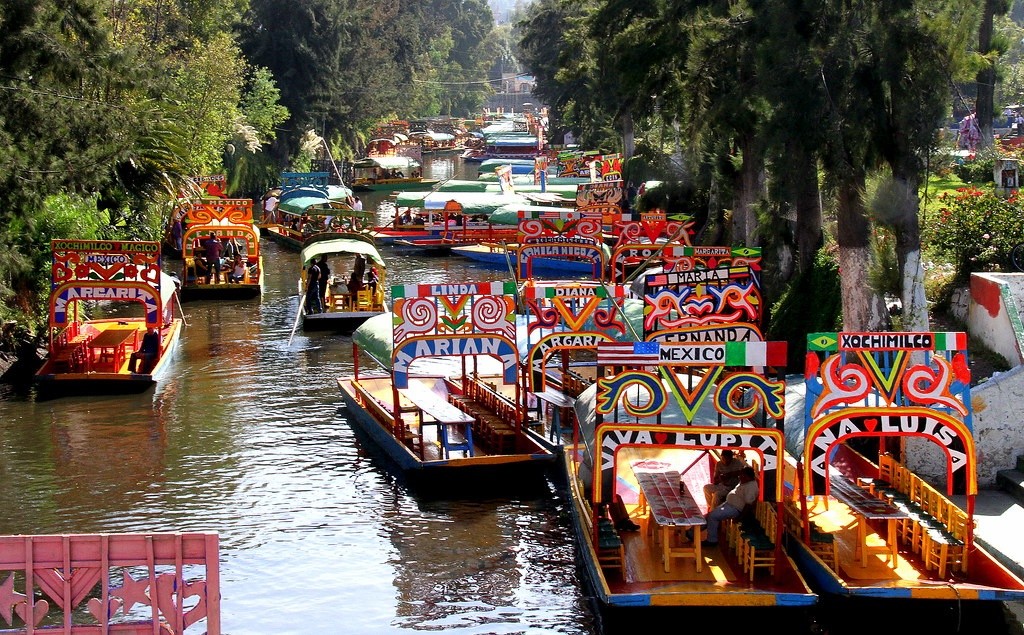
87;329;139;374
628;459;706;573
329;284;351;313
388;379;476;460
814;475;910;568
457;235;516;244
518;380;581;445
214;268;231;285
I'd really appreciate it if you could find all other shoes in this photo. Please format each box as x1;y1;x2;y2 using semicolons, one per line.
702;539;719;547
686;529;694;540
613;519;640;532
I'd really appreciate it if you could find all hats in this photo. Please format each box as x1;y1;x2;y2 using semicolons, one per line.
742;467;754;479
722;450;733;457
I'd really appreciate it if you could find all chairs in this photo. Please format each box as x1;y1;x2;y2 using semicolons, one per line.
452;229;519;244
351;380;425;462
704;484;778;581
856;455;970;579
546;374;591;427
234;267;248;284
356;284;384;312
581;497;626;582
443;374;544;456
50;320;95;374
783;499;838;576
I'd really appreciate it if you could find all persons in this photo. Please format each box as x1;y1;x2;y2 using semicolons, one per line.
290;214;357;235
224;250;245;284
264;192;280;225
579;448;641;531
354;253;366;283
128;327;162;374
704;450;745;513
344;272;362;308
364;267;378;296
195;252;211;284
203;233;223;285
316;254;330;308
305;258;322;316
626;181;637;215
677;467;759;549
350;196;362;211
1015;114;1024;136
435;140;455;148
313;258;318;264
393;209;489;228
371;168;420;178
639;182;646;201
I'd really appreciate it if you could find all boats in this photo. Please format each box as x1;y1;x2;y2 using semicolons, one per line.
35;238;183;389
176;173;264;299
467;209;1023;635
336;284;557;478
339;111;692;278
296;233;390;326
263;170;372;245
